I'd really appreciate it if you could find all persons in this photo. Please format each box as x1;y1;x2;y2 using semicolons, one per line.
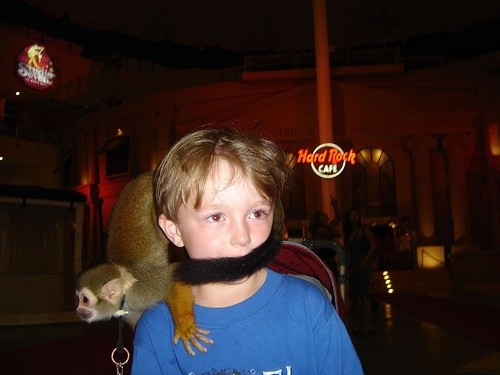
342;208;376;307
307;192;342;284
128;124;365;375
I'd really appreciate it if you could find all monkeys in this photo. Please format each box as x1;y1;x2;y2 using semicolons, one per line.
75;171;212;355
